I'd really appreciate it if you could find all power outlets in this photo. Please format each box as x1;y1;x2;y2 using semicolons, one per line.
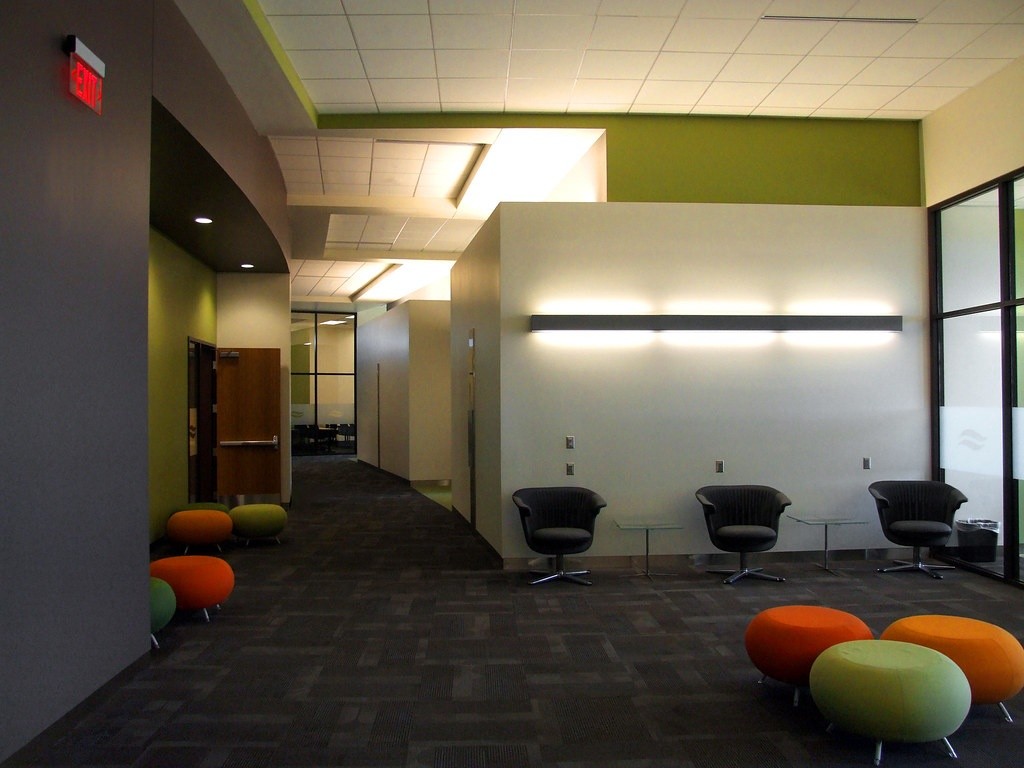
567;463;574;476
863;457;871;470
715;460;724;473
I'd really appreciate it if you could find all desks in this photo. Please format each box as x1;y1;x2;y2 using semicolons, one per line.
306;426;335;451
613;515;686;583
785;512;872;583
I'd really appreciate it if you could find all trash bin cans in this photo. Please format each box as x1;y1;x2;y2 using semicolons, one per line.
956;519;998;562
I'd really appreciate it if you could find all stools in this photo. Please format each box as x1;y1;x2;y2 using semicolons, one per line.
746;604;1024;767
151;556;235;648
170;503;289;554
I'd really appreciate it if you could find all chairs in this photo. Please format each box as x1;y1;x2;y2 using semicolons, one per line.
865;479;967;581
512;485;607;590
295;425;355;454
693;484;795;586
